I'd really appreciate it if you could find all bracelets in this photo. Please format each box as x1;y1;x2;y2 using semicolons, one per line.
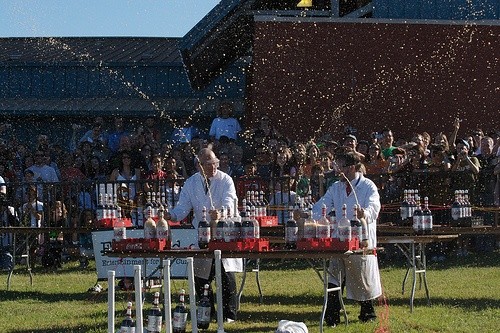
168;212;171;220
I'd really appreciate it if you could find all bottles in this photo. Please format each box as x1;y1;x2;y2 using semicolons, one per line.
351;204;362;247
156;210;168;241
114;206;127;242
144;206;156;238
329;208;338;238
338;204;351;241
401;189;433;236
147;292;162;333
197;284;211;330
357;204;368;247
96;193;121;219
284;193;316;251
316;204;330;238
198;190;266;250
144;192;169;218
120;302;136;333
173;289;188;333
452;190;471;222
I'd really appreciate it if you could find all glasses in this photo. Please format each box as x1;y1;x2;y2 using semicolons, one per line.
36;154;44;157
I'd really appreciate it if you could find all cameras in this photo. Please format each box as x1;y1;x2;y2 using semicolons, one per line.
433;145;445;155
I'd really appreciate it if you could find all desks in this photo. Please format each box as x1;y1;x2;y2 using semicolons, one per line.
0;202;500;333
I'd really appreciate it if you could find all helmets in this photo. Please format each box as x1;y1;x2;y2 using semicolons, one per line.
194;148;220;165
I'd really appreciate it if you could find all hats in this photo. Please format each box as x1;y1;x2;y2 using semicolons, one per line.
344;134;357;142
455;137;470;149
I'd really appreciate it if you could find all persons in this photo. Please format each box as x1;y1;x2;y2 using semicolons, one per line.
0;101;500;269
294;152;380;327
155;148;243;323
